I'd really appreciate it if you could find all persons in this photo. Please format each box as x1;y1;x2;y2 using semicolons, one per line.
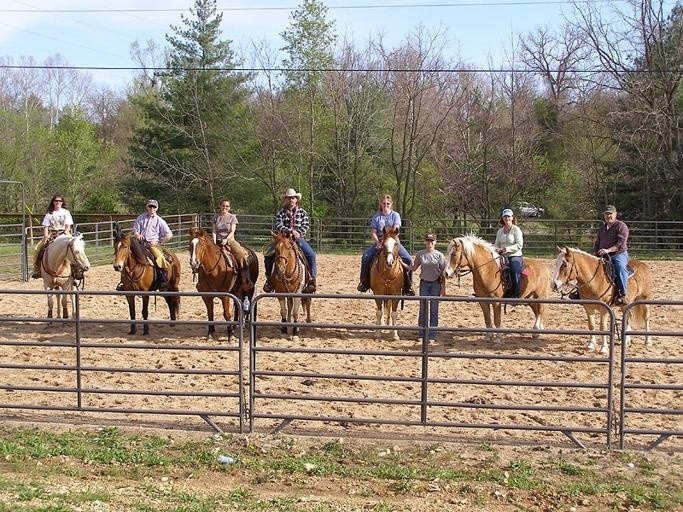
357;194;415;296
32;194;84;280
569;204;629;306
398;233;446;343
115;199;173;291
472;208;523;305
210;197;251;293
263;188;317;293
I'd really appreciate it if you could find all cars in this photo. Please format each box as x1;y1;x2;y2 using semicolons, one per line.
519;202;544;218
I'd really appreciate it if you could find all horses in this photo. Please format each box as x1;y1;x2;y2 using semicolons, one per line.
32;232;90;330
113;230;181;335
369;226;405;341
550;245;653;355
444;228;550;345
270;230;313;338
189;228;259;343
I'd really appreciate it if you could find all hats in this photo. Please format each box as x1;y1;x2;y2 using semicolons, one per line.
501;209;513;217
601;205;616;214
145;199;158;209
424;233;437;241
280;188;302;203
